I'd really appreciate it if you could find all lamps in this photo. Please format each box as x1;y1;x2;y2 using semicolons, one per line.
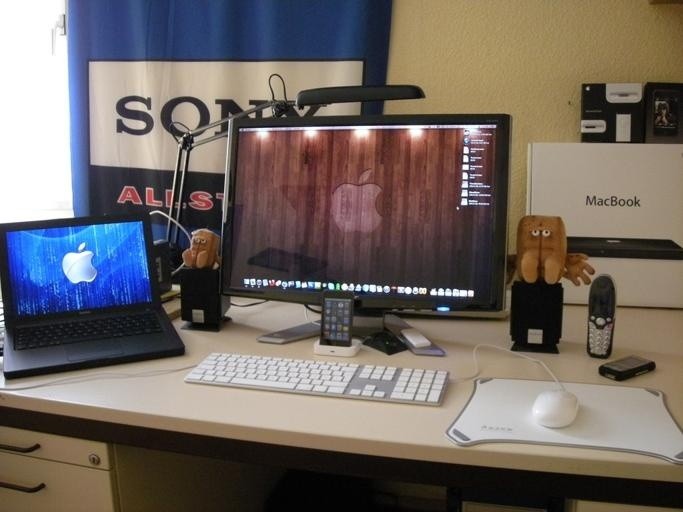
152;74;425;269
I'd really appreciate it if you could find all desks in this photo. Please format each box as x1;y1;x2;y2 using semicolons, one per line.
0;293;683;511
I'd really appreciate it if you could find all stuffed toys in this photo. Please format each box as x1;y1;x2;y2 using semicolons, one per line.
182;228;221;268
516;215;594;287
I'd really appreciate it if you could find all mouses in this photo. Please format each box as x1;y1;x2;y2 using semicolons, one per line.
531;389;579;429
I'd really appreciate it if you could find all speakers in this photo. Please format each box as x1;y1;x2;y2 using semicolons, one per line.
510;281;562;354
180;266;230;330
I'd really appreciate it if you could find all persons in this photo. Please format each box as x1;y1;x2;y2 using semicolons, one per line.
655;102;679;128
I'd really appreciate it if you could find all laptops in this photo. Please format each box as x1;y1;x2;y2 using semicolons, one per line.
565;236;682;261
0;210;185;379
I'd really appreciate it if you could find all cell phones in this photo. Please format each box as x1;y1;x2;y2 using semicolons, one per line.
600;355;655;381
320;291;354;346
652;89;676;137
586;275;615;359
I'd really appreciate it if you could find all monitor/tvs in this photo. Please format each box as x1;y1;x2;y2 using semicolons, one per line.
219;114;511;355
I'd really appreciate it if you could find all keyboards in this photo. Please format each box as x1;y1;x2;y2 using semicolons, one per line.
185;352;450;405
